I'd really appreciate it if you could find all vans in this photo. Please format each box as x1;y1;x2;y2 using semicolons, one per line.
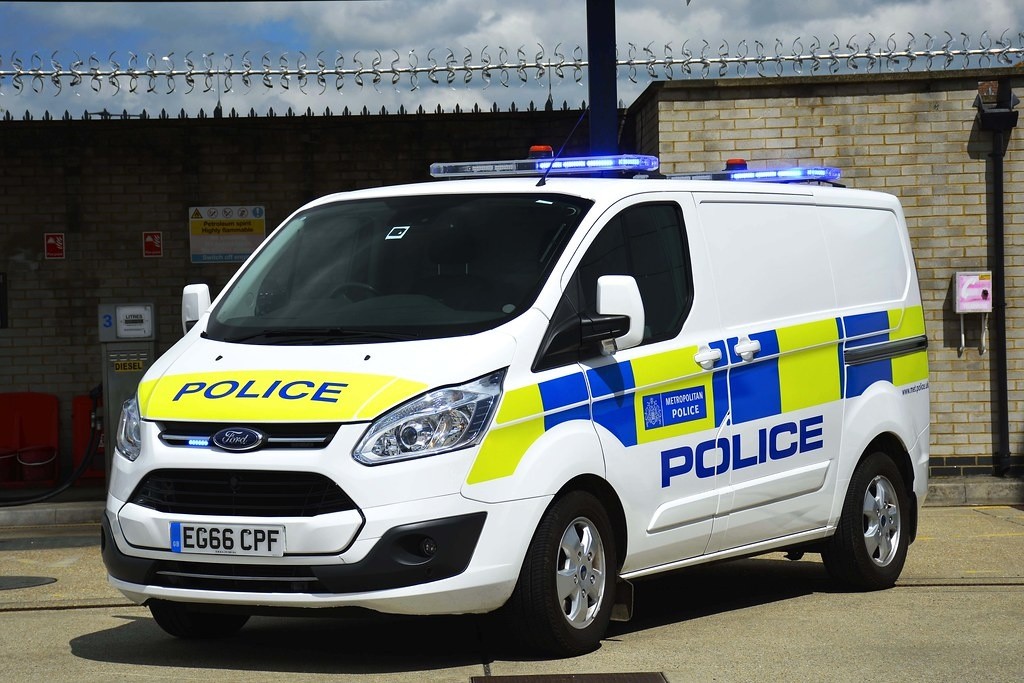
102;178;929;657
